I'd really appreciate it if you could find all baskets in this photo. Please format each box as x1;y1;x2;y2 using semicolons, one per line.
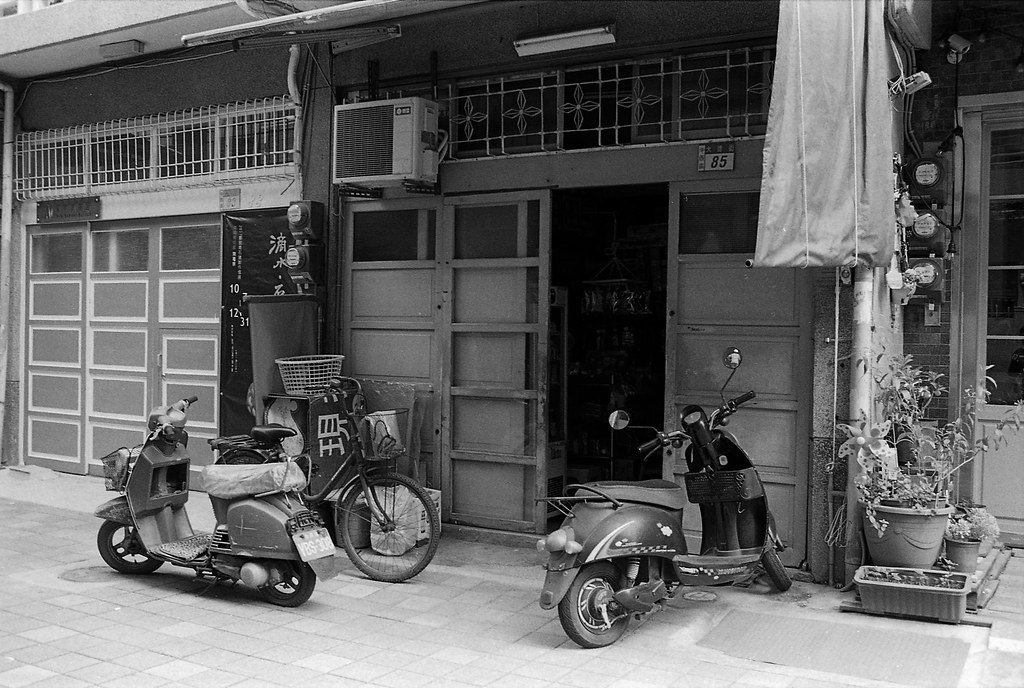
351;408;410;460
274;355;344;396
100;443;145;491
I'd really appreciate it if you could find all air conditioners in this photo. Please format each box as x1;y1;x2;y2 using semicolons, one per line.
332;97;440;194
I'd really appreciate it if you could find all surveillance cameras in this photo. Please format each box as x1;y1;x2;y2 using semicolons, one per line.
944;34;972;55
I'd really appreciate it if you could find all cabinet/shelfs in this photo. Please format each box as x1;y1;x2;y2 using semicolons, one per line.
547;286;645;519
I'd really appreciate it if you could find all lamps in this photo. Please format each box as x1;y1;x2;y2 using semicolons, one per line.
512;22;618;57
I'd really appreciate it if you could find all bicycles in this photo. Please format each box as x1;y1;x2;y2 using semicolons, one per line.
208;376;440;581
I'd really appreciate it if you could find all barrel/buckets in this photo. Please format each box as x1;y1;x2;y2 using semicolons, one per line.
332;501;372;548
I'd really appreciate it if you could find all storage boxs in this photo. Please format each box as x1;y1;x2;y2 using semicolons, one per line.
568;466;600;484
335;485;443;551
615;459;637;481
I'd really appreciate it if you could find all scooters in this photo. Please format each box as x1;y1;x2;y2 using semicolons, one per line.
94;395;338;607
533;347;792;648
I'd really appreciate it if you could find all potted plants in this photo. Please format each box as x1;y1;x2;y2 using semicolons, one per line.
853;566;971;625
834;339;1024;567
944;500;1001;573
